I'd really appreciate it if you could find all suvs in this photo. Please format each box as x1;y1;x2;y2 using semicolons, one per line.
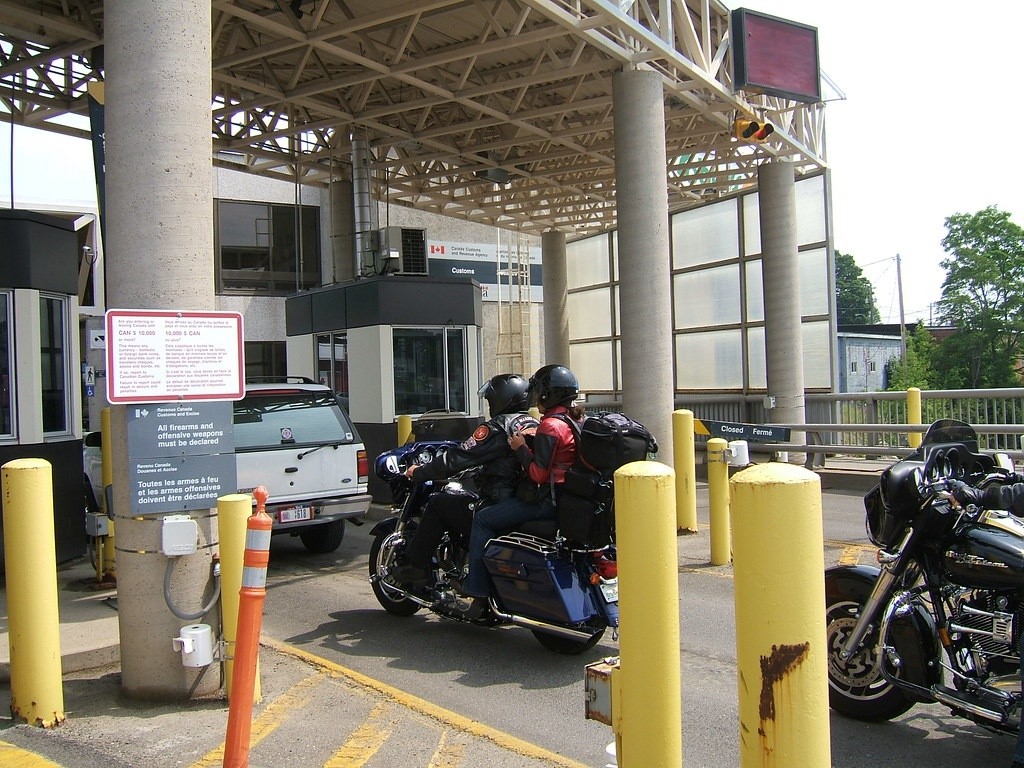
83;375;372;556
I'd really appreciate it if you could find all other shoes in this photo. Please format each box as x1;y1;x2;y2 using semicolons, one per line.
453;602;488;617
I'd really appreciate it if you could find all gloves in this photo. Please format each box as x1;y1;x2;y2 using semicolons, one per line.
952;485;984;508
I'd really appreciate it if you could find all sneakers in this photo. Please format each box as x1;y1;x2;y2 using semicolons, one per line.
392;564;432;586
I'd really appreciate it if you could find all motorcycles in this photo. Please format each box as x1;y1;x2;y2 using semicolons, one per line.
366;407;623;657
824;416;1023;746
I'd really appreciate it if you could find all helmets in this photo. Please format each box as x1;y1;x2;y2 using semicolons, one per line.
484;374;529;418
594;559;618;579
534;364;579;409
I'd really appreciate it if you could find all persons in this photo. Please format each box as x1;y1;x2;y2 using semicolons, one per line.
953;474;1024;518
391;373;539;586
451;365;593;597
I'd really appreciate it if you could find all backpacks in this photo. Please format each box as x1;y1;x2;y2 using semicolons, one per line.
546;410;658;548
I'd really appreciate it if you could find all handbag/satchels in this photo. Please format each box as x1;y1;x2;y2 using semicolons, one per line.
515;477;550;505
